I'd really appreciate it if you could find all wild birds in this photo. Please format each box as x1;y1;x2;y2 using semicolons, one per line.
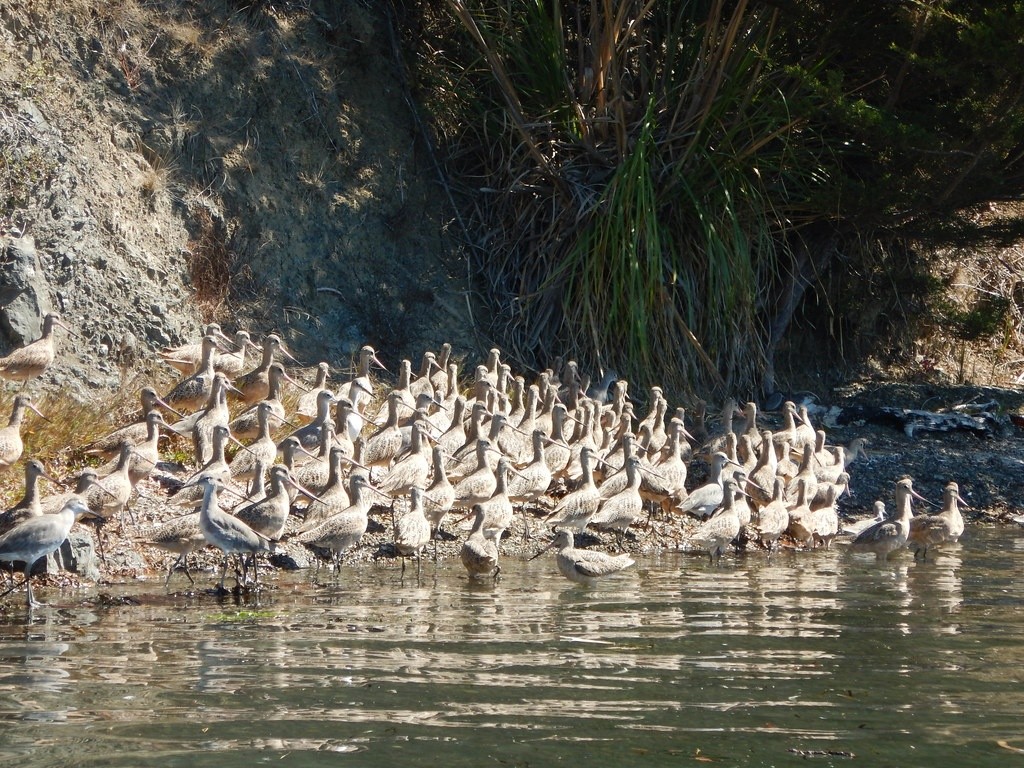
0;312;966;607
